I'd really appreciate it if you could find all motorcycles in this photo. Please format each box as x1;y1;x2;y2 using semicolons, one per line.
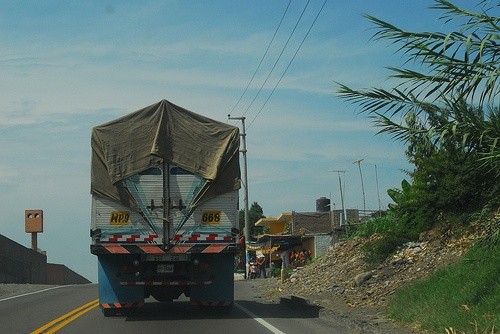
247;263;257;279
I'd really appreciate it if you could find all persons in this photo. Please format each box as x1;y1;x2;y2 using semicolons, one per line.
256;254;268;278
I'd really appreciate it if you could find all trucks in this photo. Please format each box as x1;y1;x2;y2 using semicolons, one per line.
86;98;243;315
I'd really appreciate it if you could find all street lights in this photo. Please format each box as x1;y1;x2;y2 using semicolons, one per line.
333;169;348;225
352;157;370;215
